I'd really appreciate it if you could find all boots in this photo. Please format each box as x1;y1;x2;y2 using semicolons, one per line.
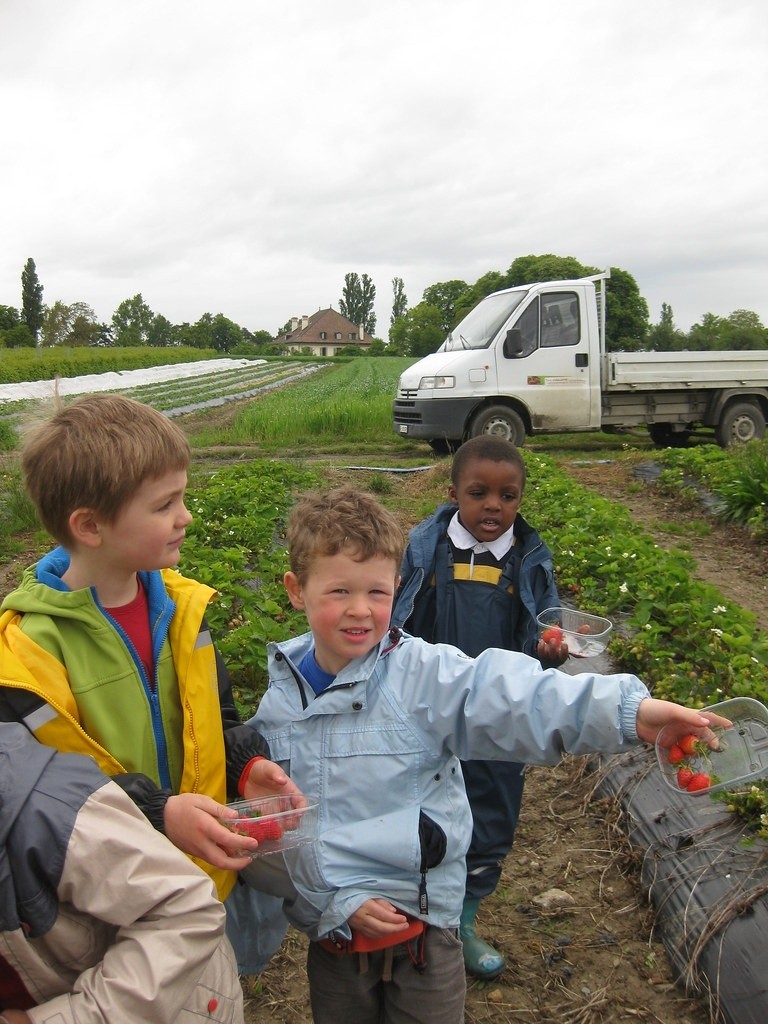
459;896;507;978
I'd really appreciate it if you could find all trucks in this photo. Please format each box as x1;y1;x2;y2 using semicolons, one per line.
392;271;768;458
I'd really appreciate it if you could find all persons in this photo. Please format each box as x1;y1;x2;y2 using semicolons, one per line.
386;431;593;984
2;391;309;1023
0;670;248;1024
217;482;732;1022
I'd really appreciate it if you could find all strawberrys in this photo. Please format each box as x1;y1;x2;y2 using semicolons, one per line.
669;734;713;794
541;622;564;645
224;805;283;851
